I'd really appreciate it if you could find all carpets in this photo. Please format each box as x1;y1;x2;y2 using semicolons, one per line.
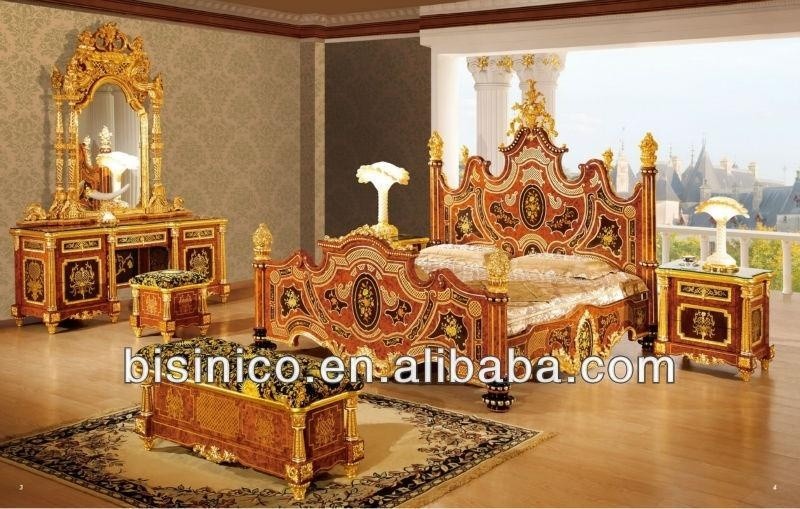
0;392;563;509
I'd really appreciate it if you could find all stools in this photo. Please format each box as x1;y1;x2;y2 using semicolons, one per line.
127;268;214;345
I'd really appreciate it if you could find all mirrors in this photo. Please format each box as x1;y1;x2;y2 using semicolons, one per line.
51;18;168;223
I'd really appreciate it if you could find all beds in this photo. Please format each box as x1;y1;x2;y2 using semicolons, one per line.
253;71;661;407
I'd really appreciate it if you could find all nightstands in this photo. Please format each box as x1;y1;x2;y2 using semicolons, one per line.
652;257;777;381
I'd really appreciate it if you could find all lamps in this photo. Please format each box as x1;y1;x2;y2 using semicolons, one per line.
95;151;141;201
693;197;750;274
354;160;412;242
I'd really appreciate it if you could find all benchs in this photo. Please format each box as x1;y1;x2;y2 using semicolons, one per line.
132;336;367;502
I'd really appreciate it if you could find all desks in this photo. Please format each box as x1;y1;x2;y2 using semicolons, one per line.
8;214;231;334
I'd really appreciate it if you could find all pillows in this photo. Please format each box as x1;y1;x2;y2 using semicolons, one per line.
420;243;620;280
